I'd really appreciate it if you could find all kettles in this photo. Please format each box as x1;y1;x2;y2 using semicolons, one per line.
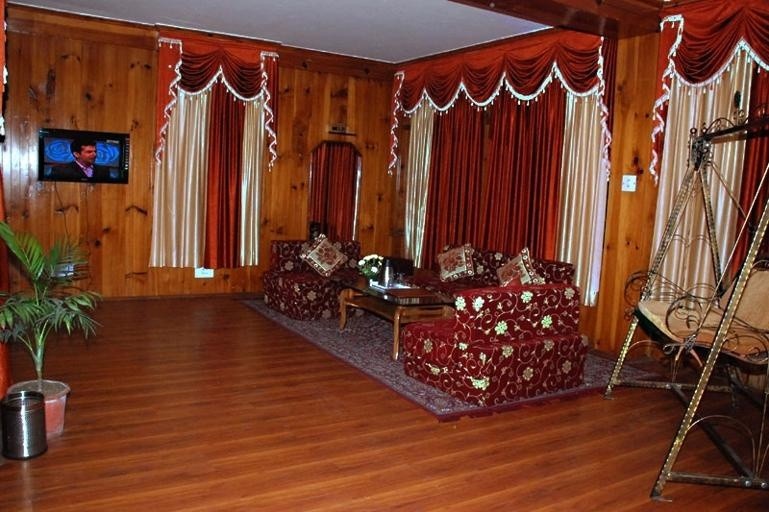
378;259;394;286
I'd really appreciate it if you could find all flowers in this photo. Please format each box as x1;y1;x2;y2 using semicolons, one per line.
358;254;384;280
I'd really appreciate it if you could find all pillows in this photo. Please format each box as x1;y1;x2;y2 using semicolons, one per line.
297;233;350;277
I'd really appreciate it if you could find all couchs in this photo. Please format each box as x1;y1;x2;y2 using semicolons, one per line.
402;242;589;407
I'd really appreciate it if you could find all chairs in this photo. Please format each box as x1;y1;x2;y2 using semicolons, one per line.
261;240;360;323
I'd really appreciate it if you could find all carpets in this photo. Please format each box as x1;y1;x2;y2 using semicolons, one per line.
239;295;662;423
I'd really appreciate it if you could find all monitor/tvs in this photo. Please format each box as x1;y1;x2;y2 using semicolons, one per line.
38;127;130;185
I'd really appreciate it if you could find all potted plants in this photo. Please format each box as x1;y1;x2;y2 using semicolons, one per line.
1;219;105;441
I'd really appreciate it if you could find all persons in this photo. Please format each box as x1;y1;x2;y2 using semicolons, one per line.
47;138;112;178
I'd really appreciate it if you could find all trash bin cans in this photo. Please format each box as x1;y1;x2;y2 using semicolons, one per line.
0;391;47;460
